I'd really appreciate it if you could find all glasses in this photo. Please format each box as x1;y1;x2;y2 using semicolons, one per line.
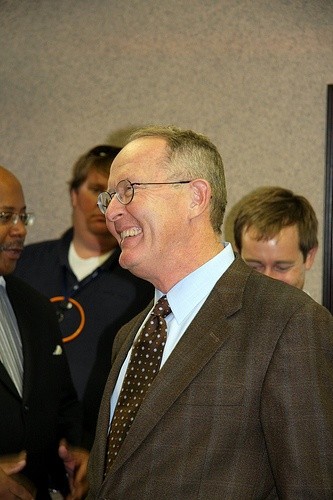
0;211;36;227
96;179;215;215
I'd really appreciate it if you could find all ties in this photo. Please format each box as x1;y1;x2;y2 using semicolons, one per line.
103;294;172;483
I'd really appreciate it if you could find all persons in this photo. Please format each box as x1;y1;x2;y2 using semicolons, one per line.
0;166;93;500
15;144;155;454
234;188;319;291
84;125;333;500
